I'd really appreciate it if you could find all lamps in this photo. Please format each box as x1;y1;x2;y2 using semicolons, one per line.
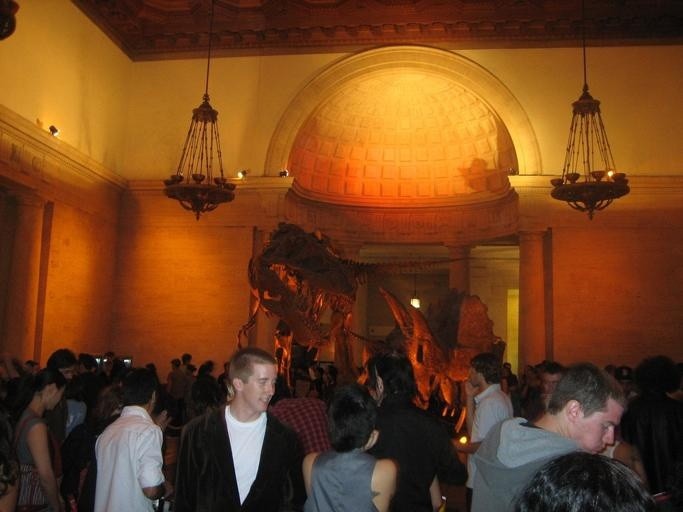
49;125;59;138
552;2;630;223
238;171;247;179
164;0;237;221
280;171;289;178
410;274;421;309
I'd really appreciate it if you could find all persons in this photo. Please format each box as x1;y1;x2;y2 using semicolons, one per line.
0;348;174;511
450;353;682;511
172;348;448;511
